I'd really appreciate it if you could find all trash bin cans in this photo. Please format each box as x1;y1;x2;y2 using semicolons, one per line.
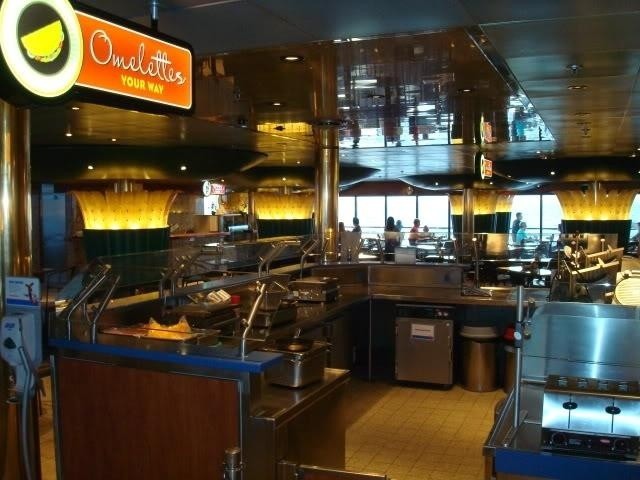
459;323;516;394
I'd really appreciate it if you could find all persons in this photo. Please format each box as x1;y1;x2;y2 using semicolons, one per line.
384;217;403;260
632;222;640;241
352;217;361;232
408;218;420;245
512;212;527;245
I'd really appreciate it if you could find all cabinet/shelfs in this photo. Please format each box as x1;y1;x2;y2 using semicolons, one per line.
395;304;455;389
302;310;355;371
